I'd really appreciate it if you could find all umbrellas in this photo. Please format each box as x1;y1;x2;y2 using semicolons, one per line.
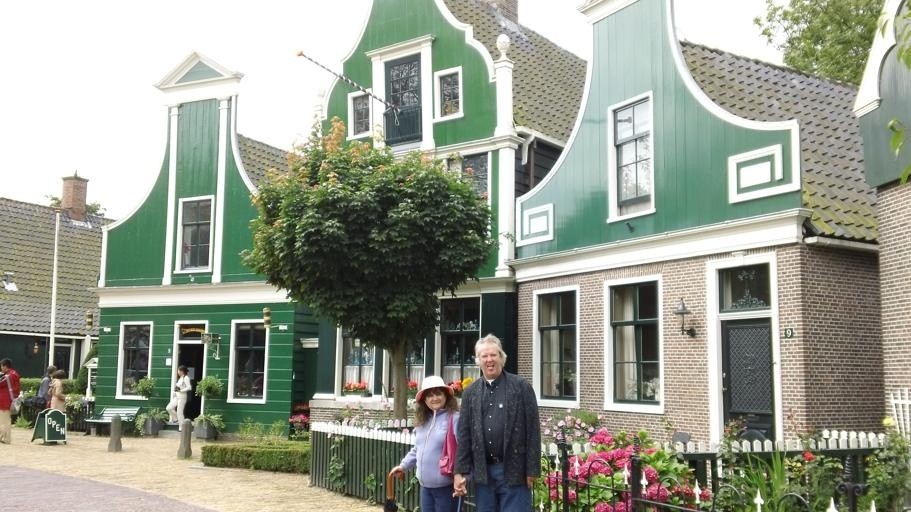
384;467;404;512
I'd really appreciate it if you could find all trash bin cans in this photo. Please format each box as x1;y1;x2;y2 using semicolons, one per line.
32;396;46;428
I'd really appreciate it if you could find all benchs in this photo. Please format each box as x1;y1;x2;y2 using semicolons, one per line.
83;406;143;434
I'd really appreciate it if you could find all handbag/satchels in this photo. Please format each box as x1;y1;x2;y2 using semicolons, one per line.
31;396;47;410
9;398;22;415
439;430;459;478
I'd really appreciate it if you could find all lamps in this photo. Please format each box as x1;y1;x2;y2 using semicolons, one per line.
262;307;282;329
673;297;697;337
85;311;108;331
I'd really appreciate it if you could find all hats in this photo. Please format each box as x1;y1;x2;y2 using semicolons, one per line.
415;376;454;405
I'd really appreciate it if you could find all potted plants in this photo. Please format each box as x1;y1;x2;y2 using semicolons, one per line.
193;375;224;397
192;413;226;438
134;407;169;435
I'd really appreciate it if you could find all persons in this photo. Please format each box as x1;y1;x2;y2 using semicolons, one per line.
0;357;22;443
387;373;472;512
36;364;58;413
451;334;542;511
46;369;71;413
166;363;192;432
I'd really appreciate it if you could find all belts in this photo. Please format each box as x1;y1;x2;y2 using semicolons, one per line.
487;457;503;465
